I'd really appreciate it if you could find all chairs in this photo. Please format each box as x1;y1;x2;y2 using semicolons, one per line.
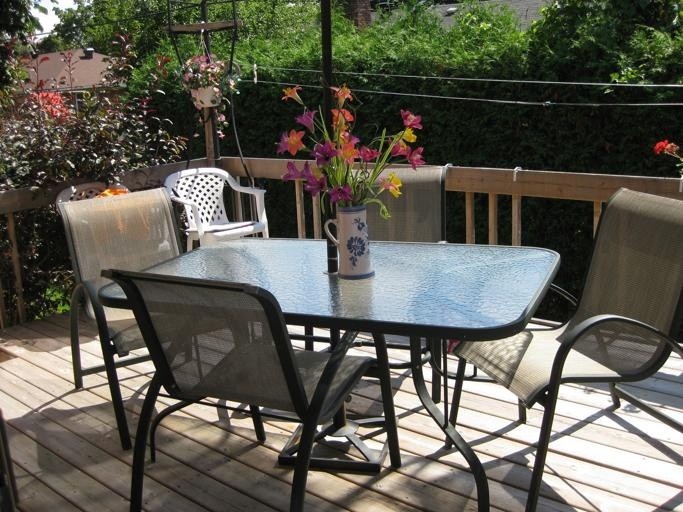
165;167;272;247
441;184;681;510
55;179;110;214
94;233;564;510
56;184;268;455
97;265;405;512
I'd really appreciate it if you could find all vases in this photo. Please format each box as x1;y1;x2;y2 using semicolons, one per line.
322;206;379;282
188;86;222;109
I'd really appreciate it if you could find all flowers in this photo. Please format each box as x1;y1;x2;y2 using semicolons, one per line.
269;78;427;220
175;54;241;139
